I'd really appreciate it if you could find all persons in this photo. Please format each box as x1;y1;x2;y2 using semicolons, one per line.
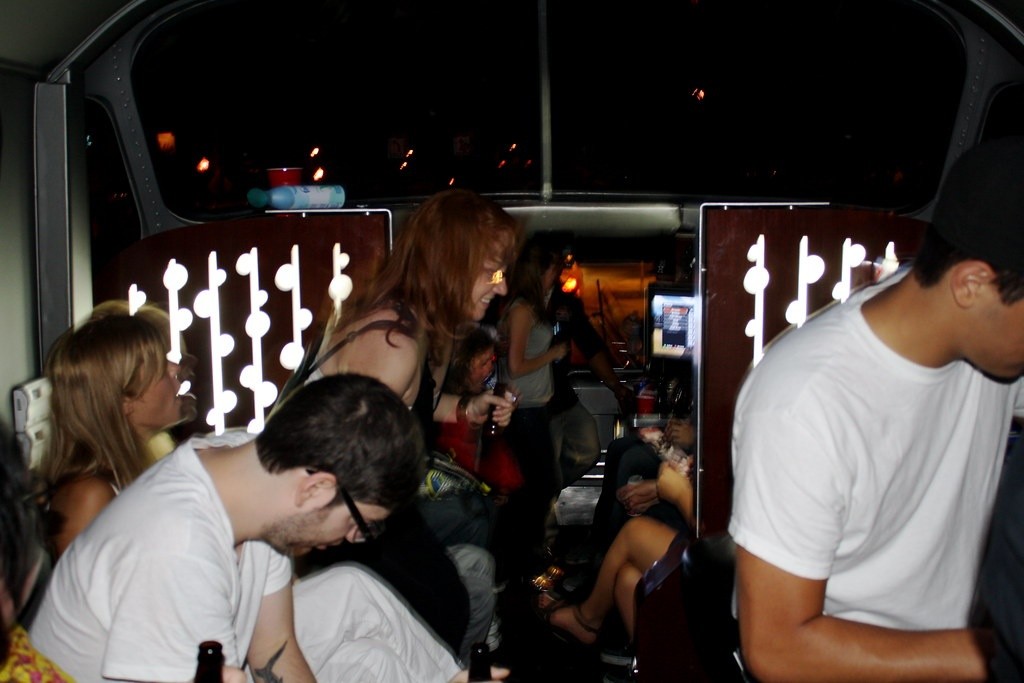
53;298;512;683
258;186;699;673
0;497;76;683
29;369;424;683
727;127;1024;683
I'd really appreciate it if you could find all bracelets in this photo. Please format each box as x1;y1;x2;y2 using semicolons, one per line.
455;395;481;433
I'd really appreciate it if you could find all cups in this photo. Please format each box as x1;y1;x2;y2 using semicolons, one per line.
639;389;658;409
267;167;303;217
637;394;654;415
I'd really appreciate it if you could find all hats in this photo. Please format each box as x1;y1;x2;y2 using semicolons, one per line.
933;137;1024;274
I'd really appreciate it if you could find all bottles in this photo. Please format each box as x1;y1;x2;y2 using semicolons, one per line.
469;643;492;682
247;185;345;208
481;383;506;461
195;640;225;682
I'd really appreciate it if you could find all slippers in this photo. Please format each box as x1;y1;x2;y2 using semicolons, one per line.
533;591;590;652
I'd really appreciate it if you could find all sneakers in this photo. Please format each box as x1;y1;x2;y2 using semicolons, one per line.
484;632;503;652
603;670;636;683
600;640;632;665
487;611;501;635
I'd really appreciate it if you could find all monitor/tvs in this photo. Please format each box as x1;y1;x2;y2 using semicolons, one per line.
646;284;696;361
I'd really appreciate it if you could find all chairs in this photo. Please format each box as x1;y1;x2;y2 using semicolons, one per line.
555;387;617;525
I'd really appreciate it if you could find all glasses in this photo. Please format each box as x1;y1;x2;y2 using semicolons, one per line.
306;469;383;544
